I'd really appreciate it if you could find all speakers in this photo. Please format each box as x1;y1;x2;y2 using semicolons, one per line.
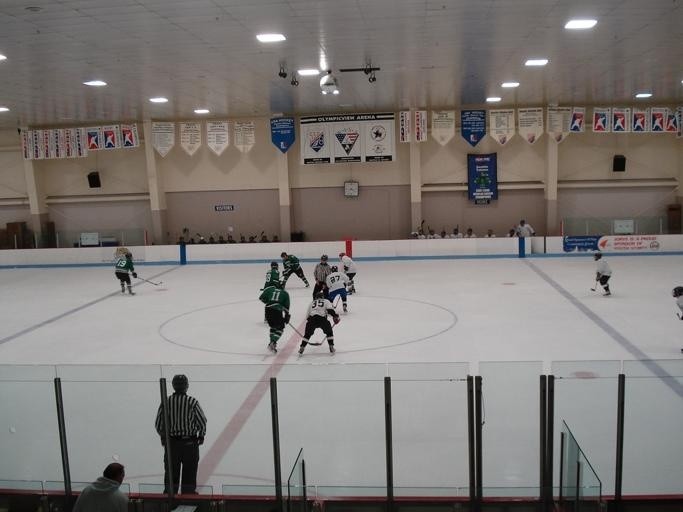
613;155;625;172
87;172;101;188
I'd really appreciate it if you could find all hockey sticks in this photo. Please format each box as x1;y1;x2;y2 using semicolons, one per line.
310;318;340;345
128;274;164;285
591;280;598;291
261;268;292;291
288;323;320;343
334;294;341;309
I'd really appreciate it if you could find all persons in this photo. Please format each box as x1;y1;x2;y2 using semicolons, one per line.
176;233;280;245
259;252;358;355
408;227;496;239
114;250;137;298
671;284;683;356
593;251;613;296
155;373;207;494
70;463;132;510
505;220;537;237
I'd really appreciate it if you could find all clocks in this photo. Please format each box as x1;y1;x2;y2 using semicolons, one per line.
343;181;358;198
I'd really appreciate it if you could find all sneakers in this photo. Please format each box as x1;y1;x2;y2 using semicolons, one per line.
343;305;347;312
328;345;335;352
298;346;304;354
266;341;277;353
345;289;354;295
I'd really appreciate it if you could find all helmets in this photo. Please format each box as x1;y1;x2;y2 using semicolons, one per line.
315;253;345;298
172;375;188;390
594;253;600;260
270;252;286;289
125;253;132;259
671;287;682;297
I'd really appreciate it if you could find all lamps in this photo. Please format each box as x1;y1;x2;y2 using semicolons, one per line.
278;60;380;95
